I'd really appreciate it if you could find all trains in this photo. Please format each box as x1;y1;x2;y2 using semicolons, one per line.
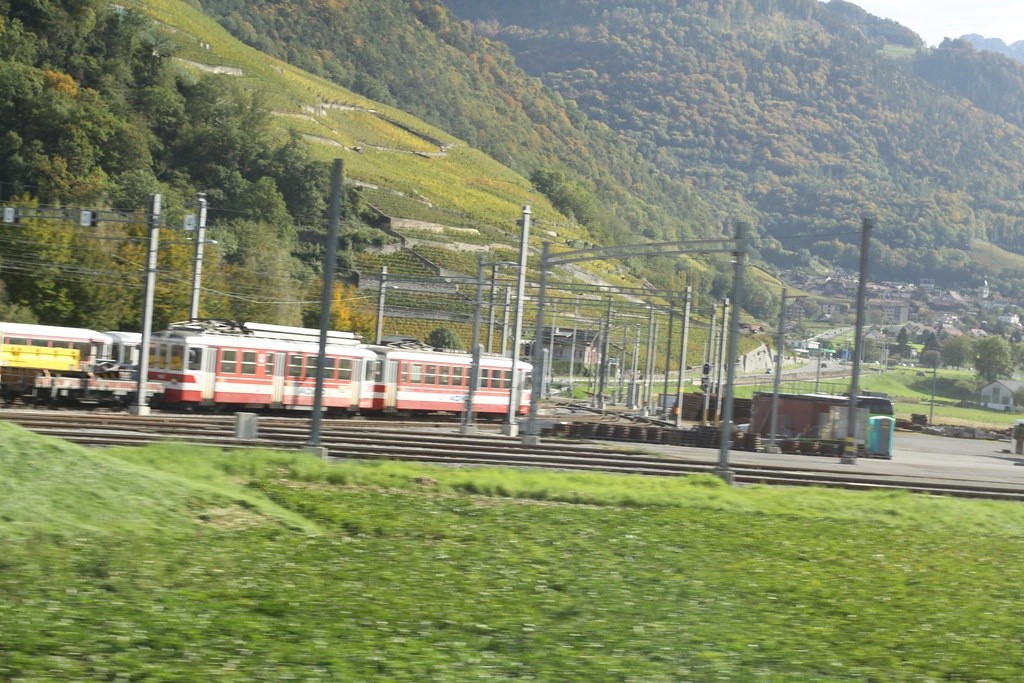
0;319;533;422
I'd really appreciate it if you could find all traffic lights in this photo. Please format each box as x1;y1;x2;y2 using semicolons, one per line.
703;364;709;375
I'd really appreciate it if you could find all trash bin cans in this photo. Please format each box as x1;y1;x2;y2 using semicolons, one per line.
864;416;895;460
